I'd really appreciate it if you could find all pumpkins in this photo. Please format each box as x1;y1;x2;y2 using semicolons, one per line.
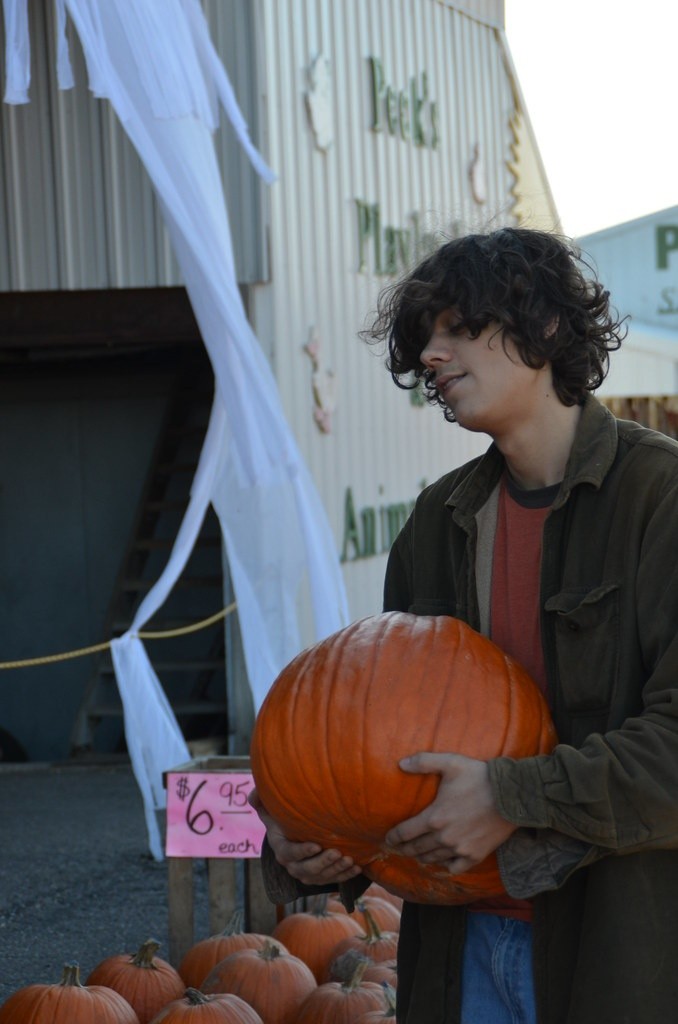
251;611;557;905
0;876;407;1024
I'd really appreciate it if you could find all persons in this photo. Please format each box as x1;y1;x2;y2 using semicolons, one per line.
258;230;678;1024
